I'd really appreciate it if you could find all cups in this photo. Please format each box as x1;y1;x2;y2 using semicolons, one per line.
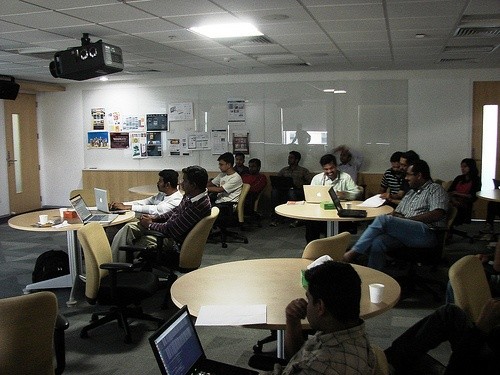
344;202;351;209
60;208;67;218
39;215;48;224
369;283;384;304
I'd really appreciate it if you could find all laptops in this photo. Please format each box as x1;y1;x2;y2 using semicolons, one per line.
302;184;338;203
68;193;119;224
147;305;259;375
328;185;366;217
93;187;131;213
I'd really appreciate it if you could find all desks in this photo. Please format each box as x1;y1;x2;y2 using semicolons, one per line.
276;201;394;237
169;258;400;362
130;185;185;196
7;210;135;308
477;191;500;202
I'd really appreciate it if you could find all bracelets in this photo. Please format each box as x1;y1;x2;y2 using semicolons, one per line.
389;198;392;201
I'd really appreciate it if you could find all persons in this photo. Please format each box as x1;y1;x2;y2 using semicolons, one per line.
111;165;211;272
327;144;362;185
384;253;500;375
379;150;420;204
106;168;183;246
446;158;482;226
233;153;250;177
365;151;404;208
282;261;377;375
94;136;107;147
306;154;363;244
287;122;311;166
206;151;242;212
335;160;449;271
270;151;312;228
239;158;267;210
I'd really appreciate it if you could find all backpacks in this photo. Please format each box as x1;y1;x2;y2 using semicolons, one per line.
33;249;70;282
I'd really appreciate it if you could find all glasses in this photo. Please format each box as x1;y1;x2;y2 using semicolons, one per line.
406;173;416;176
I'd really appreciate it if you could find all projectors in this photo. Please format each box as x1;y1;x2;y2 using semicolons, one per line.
49;40;125;80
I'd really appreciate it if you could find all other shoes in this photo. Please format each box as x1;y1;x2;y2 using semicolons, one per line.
78;273;87;282
270;221;278;226
288;222;296;228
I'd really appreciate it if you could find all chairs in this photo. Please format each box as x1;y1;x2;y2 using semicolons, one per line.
0;173;500;375
